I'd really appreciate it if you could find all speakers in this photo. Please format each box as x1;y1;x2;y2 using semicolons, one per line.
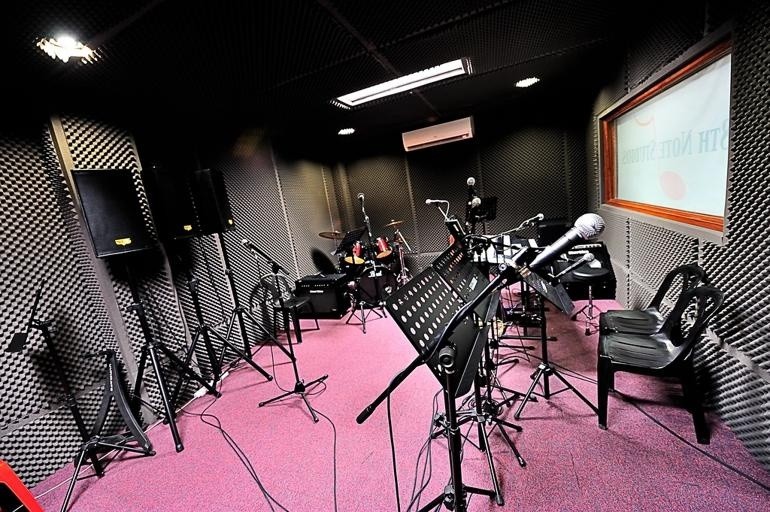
143;160;201;244
195;163;235;233
71;165;157;263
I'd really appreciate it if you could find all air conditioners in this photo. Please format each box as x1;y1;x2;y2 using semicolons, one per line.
401;115;474;153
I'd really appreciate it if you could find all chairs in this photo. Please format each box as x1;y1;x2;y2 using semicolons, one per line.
260;274;320;343
598;284;725;445
536;217;573;247
598;263;709;392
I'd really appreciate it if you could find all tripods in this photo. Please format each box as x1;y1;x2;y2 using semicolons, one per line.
339;246;383;333
111;272;223;455
26;316;157;511
356;247;600;512
212;234;297;390
247;272;330;423
162;252;273;426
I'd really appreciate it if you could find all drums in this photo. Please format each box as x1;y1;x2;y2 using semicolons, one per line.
343;257;365;276
355;260;398;304
373;236;392;258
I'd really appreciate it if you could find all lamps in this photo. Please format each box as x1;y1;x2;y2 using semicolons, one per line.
512;67;542;90
336;121;357;136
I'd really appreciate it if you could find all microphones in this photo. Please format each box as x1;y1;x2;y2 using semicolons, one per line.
356;192;367;215
426;198;448;208
467;196;481;231
498;212;605;286
241;239;291;274
467;177;476;213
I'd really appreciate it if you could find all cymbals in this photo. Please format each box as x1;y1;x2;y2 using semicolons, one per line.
319;231;346;239
384;220;405;227
